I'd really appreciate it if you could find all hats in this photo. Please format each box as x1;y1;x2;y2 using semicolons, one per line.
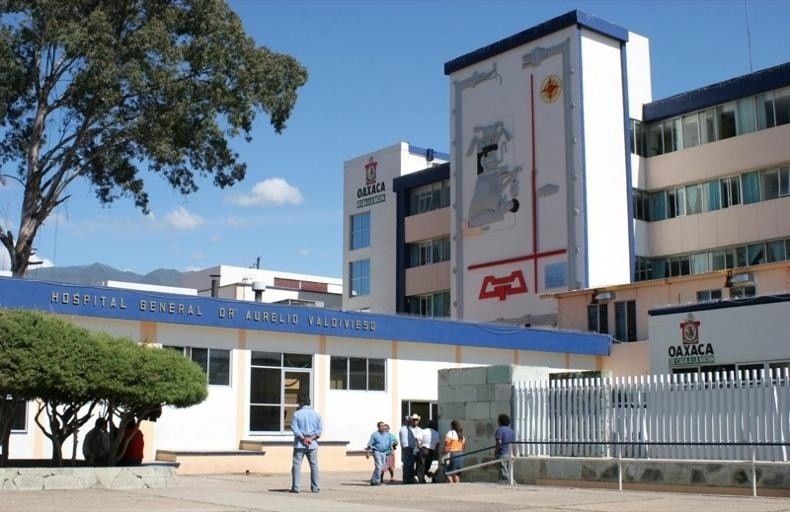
410;413;421;423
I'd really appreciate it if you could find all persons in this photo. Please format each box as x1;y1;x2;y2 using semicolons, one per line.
82;418;111;466
113;411;144;465
494;413;517;486
365;413;467;487
289;394;324;494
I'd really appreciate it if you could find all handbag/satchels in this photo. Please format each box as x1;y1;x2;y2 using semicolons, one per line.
440;451;450;465
